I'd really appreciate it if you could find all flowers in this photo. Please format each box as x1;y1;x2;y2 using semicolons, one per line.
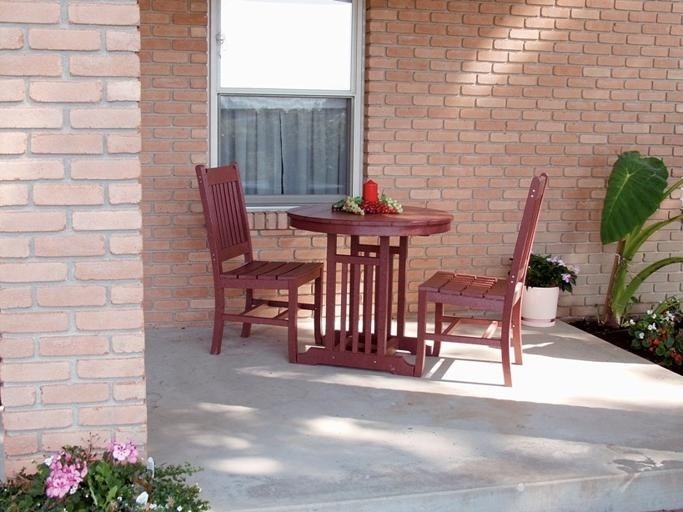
507;252;581;296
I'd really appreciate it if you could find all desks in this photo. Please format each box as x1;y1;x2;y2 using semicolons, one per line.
286;203;456;377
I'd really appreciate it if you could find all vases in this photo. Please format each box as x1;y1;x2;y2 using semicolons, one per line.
520;285;561;327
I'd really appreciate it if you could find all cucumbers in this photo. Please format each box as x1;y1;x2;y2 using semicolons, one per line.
333;201;343;209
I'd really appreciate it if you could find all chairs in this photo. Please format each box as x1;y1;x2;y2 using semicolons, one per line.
194;160;326;364
413;172;549;385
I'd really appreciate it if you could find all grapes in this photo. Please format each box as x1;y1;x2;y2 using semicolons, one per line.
343;200;364;216
383;197;402;214
359;201;396;214
